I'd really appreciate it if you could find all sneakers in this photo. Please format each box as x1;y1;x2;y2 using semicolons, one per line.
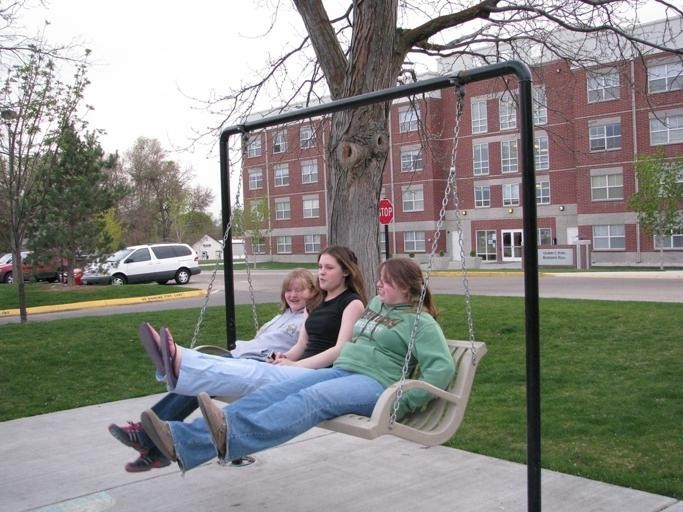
124;450;174;472
139;408;178;464
108;421;153;455
192;389;228;458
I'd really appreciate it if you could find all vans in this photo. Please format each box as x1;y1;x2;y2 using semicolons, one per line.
0;243;201;286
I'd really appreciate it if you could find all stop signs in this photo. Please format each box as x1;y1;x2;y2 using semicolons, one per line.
379;199;393;224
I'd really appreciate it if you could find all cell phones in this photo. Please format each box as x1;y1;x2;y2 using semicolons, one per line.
270;352;276;360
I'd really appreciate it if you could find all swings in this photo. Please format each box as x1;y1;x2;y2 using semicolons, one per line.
191;86;488;445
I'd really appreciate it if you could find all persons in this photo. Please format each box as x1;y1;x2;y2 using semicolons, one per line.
137;244;369;404
107;269;323;472
140;259;457;479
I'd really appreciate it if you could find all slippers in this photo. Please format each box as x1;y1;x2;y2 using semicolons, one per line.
158;325;179;392
136;321;167;378
